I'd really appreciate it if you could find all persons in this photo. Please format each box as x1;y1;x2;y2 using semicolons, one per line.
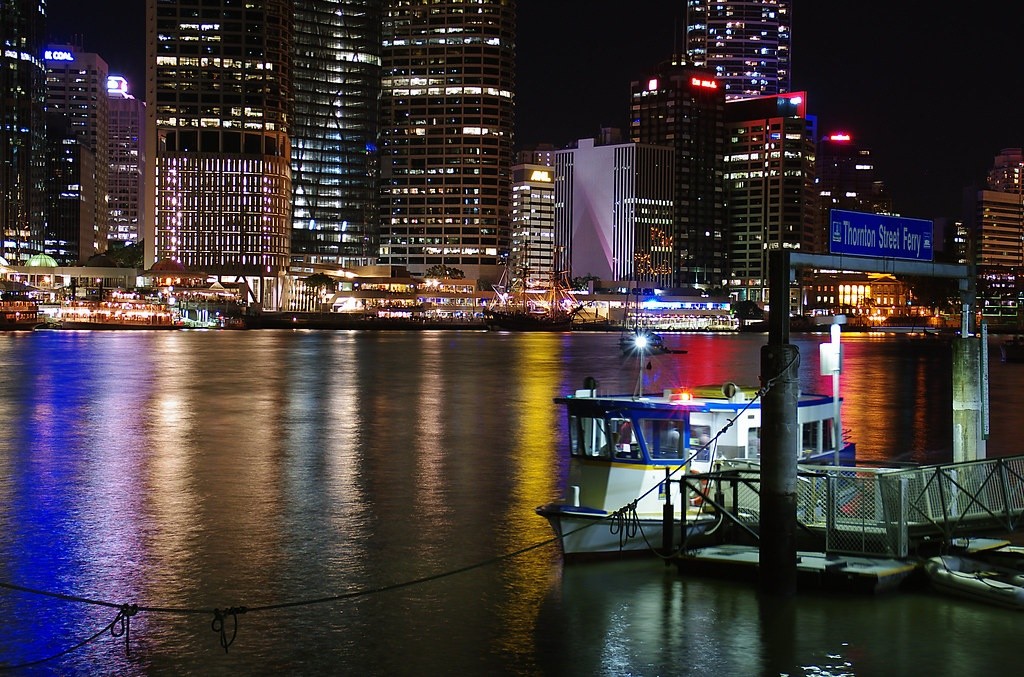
618;418;680;460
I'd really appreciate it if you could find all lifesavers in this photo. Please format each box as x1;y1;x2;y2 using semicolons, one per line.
690;470;710;507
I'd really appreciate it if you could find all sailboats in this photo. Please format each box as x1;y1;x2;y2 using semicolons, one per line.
481;235;585;332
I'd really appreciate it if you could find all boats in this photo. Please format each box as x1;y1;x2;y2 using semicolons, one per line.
1000;336;1024;363
531;376;862;559
872;325;942;334
